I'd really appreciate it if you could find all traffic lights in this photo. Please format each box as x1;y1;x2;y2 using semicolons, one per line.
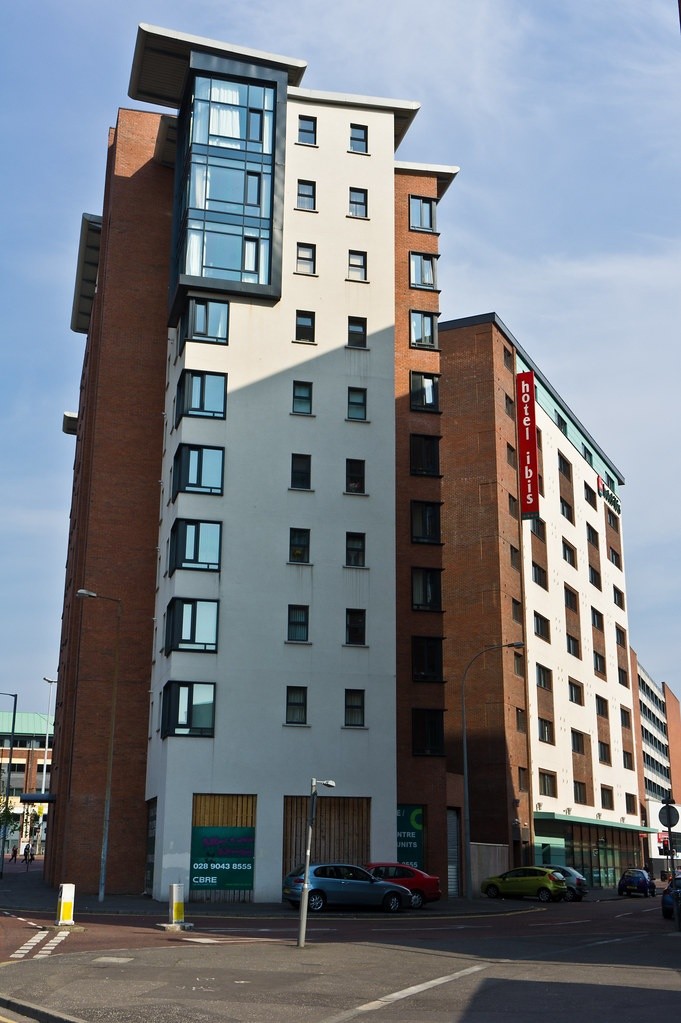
662;839;669;855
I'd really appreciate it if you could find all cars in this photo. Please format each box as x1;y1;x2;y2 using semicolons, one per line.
284;864;412;912
662;873;681;920
361;861;442;909
535;864;589;902
618;868;657;899
480;866;567;903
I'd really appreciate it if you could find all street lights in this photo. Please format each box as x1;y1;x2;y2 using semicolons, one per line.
296;777;337;946
34;676;54;873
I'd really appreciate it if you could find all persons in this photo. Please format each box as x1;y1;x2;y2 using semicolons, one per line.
27;844;35;865
9;845;18;863
21;844;30;864
